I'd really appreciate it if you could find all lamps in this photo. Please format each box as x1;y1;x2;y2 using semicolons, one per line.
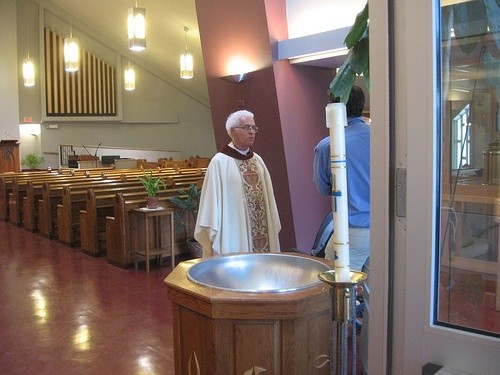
181;27;194;78
64;18;80;72
124;59;135;91
23;38;35;86
220;74;248;83
128;0;146;50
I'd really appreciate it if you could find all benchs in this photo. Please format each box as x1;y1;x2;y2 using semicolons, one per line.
0;155;213;269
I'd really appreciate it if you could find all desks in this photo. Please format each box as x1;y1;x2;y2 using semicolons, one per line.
128;207;175;272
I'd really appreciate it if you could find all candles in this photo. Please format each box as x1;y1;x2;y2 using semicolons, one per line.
325;103;350;282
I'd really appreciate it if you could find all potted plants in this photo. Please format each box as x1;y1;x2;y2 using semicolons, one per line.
137;168;165;210
170;184;204;258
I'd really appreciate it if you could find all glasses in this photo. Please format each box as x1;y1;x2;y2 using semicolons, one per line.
232;124;259;133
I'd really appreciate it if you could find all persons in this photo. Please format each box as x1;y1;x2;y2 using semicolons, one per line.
313;85;370;375
194;110;282;258
